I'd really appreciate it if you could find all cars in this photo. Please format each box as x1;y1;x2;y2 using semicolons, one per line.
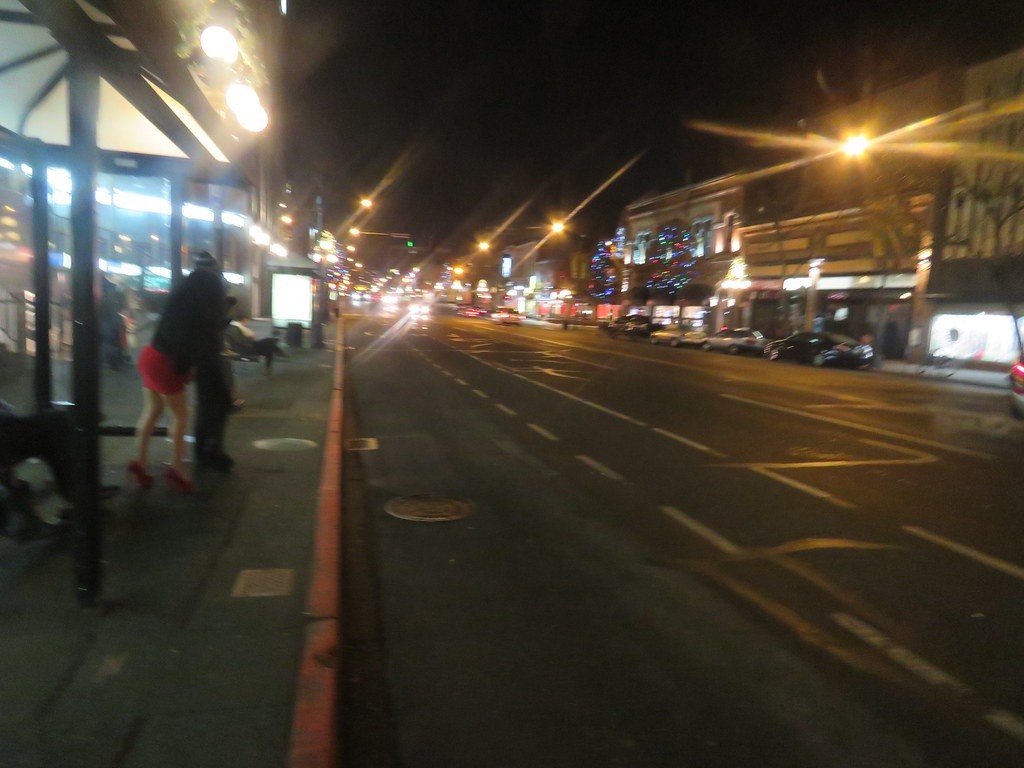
763;331;874;369
606;314;654;340
702;328;768;356
350;289;490;321
490;306;527;326
649;323;708;347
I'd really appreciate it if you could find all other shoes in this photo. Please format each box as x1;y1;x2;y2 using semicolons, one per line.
193;445;235;470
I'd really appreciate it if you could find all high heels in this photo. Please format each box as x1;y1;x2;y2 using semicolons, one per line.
127;461;152;488
164;468;196;491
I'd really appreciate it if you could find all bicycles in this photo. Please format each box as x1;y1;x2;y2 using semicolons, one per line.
917;348;959;377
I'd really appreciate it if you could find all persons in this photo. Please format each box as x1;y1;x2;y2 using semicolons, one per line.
128;249;244;489
225;314;287;378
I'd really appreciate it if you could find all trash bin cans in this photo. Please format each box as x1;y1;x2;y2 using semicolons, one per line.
288;322;302;347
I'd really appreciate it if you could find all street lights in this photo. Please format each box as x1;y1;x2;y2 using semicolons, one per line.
200;24;270;272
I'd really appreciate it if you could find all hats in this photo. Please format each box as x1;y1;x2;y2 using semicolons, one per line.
195;251;216;269
